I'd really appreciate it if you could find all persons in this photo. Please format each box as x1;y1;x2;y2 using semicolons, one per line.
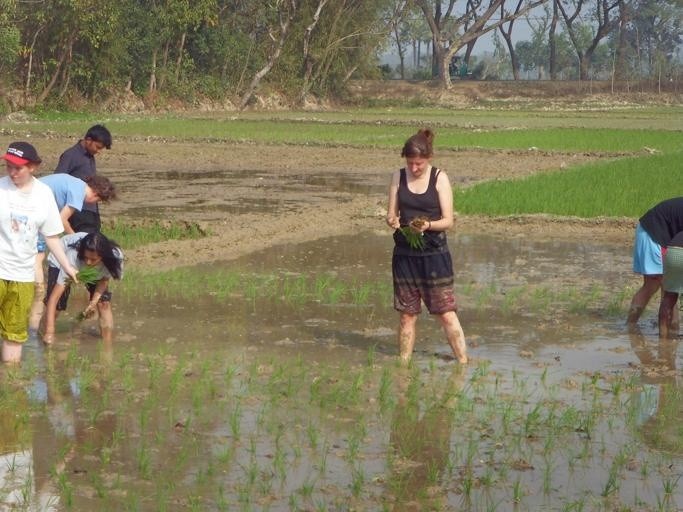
0;329;122;511
383;364;467;511
0;142;78;366
42;231;124;345
659;232;682;338
626;320;682;457
625;196;683;331
385;128;469;364
36;173;117;290
54;125;111;231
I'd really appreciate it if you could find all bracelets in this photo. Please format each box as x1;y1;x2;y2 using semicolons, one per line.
428;221;432;231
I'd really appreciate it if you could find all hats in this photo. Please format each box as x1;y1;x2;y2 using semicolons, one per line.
0;142;40;167
